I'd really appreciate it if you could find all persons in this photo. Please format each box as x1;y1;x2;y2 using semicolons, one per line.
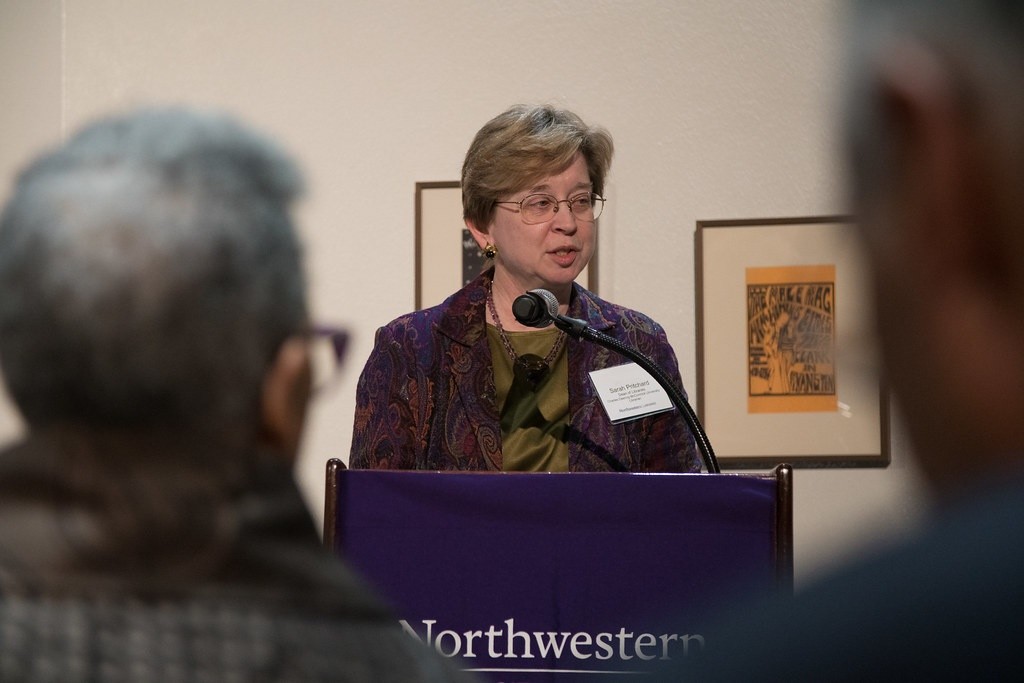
628;0;1024;683
0;103;478;683
350;105;703;473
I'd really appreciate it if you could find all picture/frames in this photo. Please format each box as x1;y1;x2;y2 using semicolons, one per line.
414;182;598;318
695;214;892;469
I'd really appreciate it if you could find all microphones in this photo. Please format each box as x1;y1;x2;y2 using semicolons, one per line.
512;288;558;329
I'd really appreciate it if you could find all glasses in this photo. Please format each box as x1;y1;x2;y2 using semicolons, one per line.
494;192;607;225
294;330;347;389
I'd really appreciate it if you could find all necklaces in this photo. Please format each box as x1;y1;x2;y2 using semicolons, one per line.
486;280;574;383
